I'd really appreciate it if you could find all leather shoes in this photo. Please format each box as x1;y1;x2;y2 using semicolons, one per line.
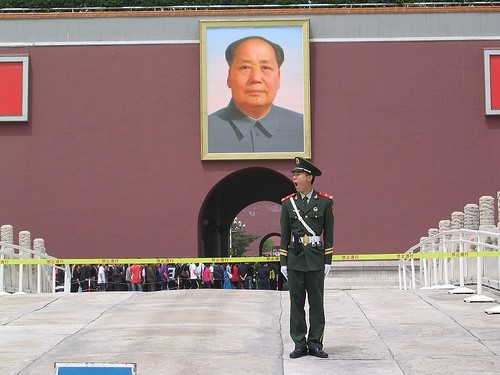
290;347;307;358
309;347;328;358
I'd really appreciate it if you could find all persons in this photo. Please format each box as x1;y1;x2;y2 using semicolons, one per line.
54;252;285;291
208;36;304;153
281;157;334;358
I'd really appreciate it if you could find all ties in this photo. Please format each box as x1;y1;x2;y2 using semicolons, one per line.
303;196;309;208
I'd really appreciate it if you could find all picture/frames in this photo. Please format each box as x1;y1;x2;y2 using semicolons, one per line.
484;47;500;115
198;17;312;161
0;54;29;122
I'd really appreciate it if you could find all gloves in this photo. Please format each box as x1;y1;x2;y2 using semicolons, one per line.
280;266;288;281
324;264;330;279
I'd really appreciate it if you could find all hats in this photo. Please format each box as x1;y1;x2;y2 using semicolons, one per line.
291;156;322;176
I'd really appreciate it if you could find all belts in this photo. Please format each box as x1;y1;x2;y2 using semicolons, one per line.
292;236;321;243
